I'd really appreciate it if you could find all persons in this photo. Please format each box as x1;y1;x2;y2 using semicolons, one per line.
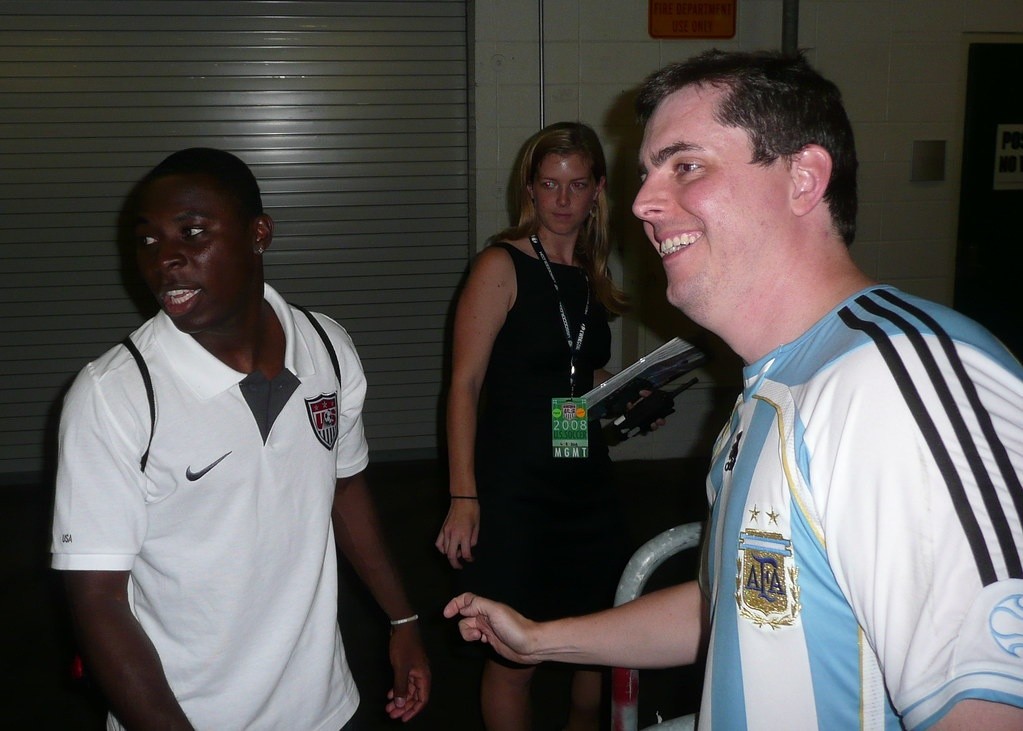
52;148;432;731
436;120;665;731
442;47;1023;731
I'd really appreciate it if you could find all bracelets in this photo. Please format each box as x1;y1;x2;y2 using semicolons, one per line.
451;495;479;500
390;614;418;625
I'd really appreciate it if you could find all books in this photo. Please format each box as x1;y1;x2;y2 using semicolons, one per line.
580;329;712;420
